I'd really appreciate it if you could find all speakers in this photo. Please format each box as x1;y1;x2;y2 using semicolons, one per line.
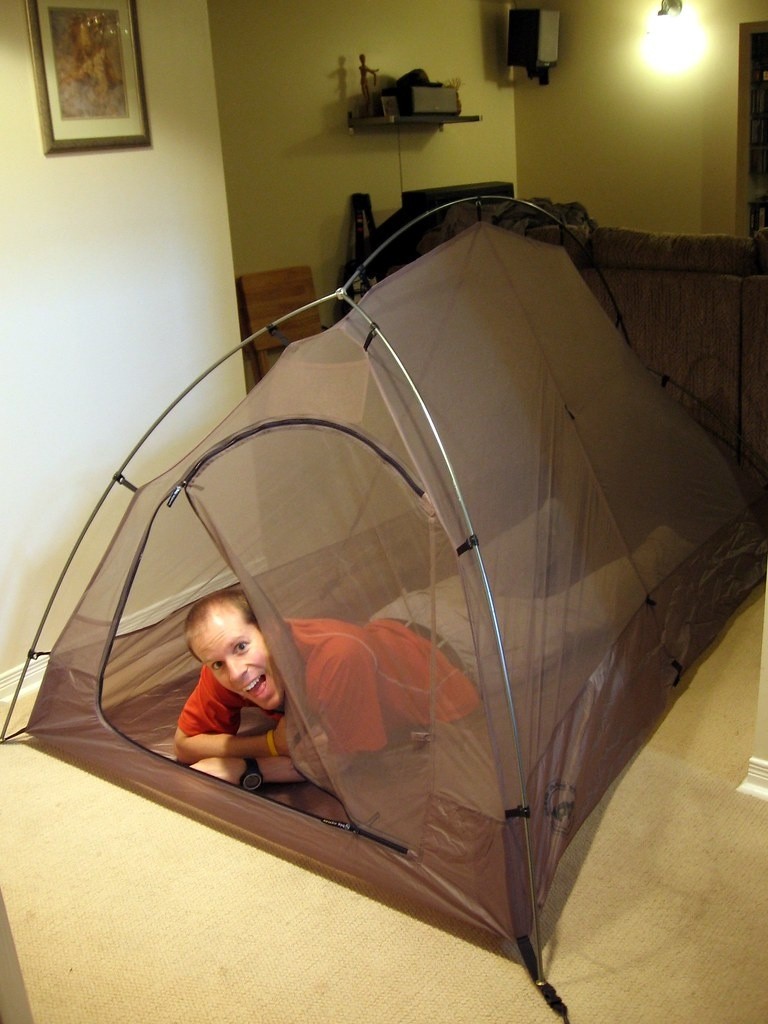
508;8;561;66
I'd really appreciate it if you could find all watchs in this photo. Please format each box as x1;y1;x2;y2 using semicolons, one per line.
239;755;263;793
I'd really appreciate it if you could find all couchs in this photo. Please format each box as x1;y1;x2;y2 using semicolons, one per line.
523;222;768;492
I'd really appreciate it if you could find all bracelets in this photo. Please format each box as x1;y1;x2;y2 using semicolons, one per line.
266;729;280;757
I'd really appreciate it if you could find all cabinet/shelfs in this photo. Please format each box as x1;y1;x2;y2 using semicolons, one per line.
748;35;768;174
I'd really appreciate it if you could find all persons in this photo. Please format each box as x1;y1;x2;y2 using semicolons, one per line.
172;487;702;793
359;53;380;117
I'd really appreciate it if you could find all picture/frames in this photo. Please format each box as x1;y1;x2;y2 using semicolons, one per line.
25;0;153;155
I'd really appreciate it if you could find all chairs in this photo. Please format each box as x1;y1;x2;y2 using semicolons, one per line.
237;264;322;384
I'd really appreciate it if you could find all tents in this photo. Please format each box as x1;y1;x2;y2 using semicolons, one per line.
3;188;768;987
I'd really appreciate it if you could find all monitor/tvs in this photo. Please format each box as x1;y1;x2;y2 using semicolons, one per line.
372;181;514;284
